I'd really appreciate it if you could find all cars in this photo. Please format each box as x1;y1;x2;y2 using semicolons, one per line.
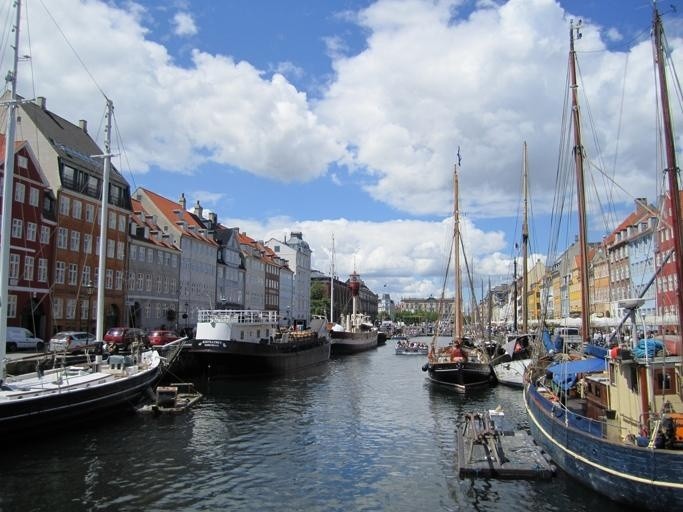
7;325;182;351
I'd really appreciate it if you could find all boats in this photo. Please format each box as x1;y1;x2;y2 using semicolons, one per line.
522;5;682;512
187;309;331;370
329;281;380;353
2;100;181;425
421;164;491;398
381;315;499;337
397;339;429;356
488;332;540;389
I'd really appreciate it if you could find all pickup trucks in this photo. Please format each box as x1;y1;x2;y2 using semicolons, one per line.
556;325;581;344
